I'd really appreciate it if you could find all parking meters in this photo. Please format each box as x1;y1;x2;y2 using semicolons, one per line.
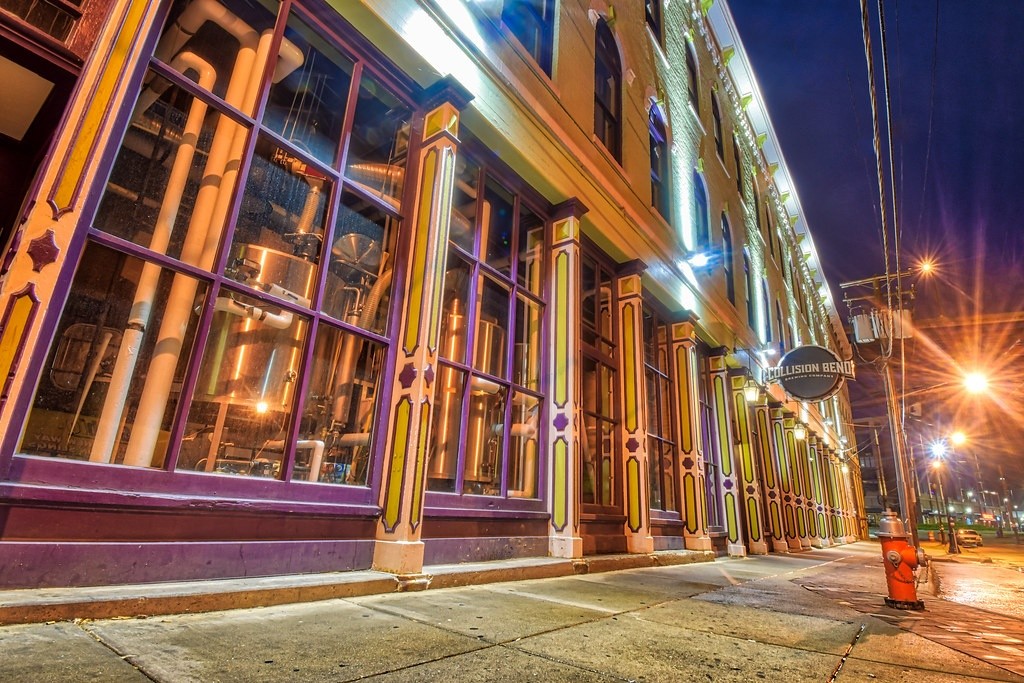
949;517;959;554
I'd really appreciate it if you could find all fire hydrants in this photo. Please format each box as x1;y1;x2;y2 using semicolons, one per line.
872;506;932;609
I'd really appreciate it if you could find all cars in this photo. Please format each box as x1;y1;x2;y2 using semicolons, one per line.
955;528;984;545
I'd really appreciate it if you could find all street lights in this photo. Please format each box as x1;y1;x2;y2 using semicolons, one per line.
909;430;966;507
838;262;935;548
